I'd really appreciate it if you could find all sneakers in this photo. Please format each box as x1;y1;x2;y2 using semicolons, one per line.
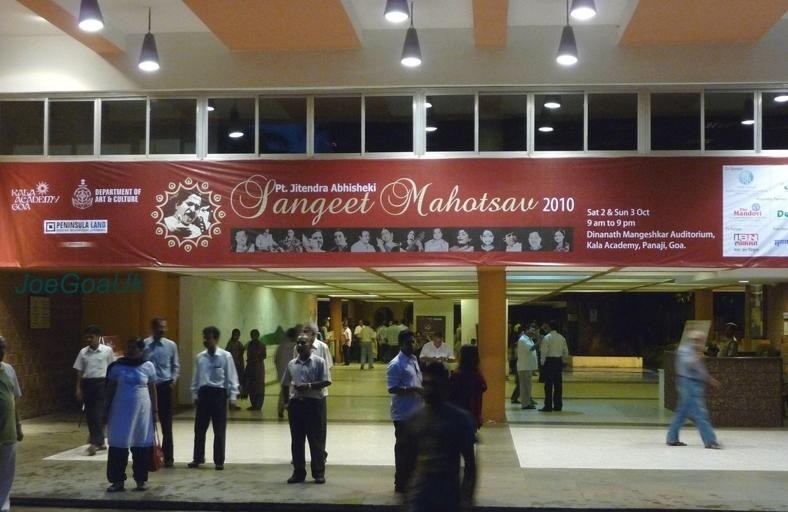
136;481;149;491
108;480;124;492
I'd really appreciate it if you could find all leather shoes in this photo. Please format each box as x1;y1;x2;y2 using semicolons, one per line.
288;476;299;483
216;463;223;469
188;459;205;468
315;477;325;483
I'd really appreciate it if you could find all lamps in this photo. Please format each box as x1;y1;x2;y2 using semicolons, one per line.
773;93;788;102
383;0;438;132
228;103;244;138
138;7;160;72
537;0;597;132
78;0;104;32
208;99;215;111
741;94;754;125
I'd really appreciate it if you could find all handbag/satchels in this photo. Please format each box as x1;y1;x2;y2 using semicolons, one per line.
149;444;166;472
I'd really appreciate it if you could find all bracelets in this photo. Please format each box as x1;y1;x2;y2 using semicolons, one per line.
16;420;22;425
153;410;158;414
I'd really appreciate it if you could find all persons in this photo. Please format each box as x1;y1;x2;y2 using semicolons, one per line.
275;320;487;505
226;329;247;399
232;228;351;252
96;335;160;492
243;330;267;410
163;190;211;241
351;228;424;252
0;337;24;511
507;319;568;412
667;330;722;448
72;323;113;455
187;327;239;470
424;227;497;252
503;229;569;252
143;317;180;467
